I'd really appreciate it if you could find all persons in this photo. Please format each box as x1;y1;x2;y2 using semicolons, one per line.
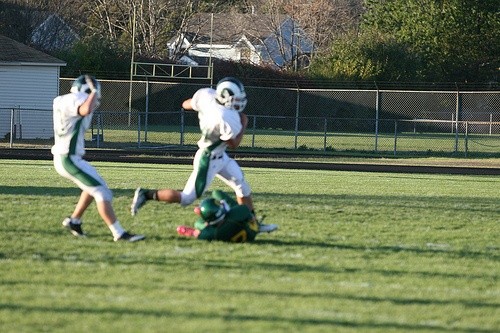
50;75;146;242
130;76;278;233
176;190;259;243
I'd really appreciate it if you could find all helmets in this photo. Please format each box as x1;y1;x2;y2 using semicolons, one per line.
70;75;101;101
215;77;247;112
200;198;226;226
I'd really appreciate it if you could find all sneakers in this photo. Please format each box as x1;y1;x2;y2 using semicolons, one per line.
62;215;88;239
113;232;145;242
194;206;201;216
259;223;277;232
177;226;196;238
131;187;148;217
